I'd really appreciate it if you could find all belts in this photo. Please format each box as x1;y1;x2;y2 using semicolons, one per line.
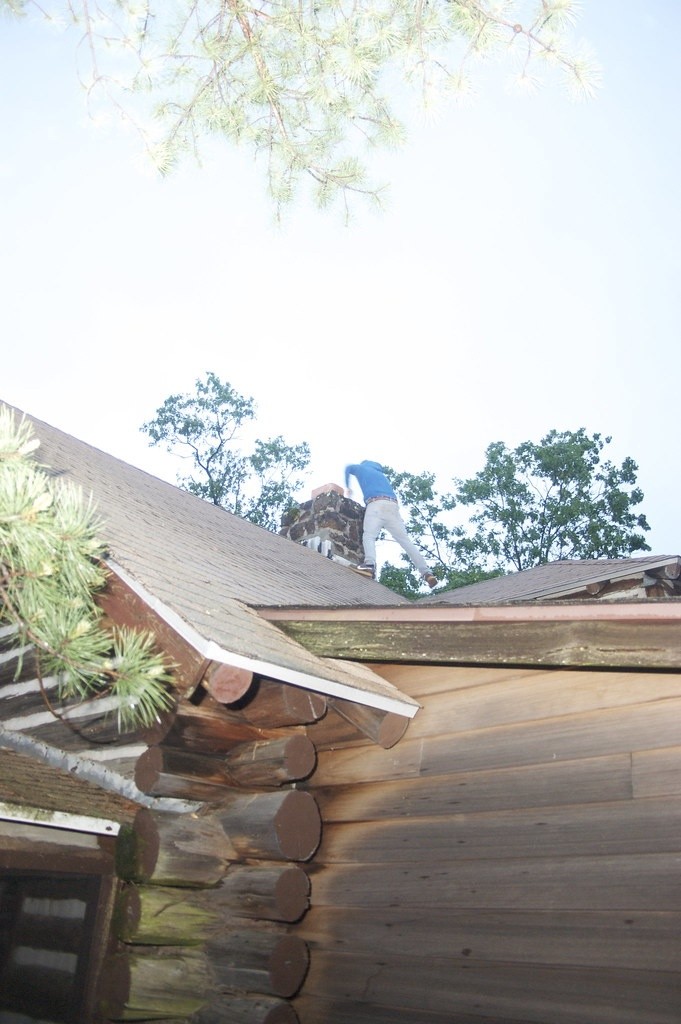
366;496;398;504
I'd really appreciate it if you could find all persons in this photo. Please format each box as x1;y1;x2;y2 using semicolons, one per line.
345;460;437;589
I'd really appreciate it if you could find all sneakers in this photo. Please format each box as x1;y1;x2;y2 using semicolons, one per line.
424;573;438;588
349;564;373;577
